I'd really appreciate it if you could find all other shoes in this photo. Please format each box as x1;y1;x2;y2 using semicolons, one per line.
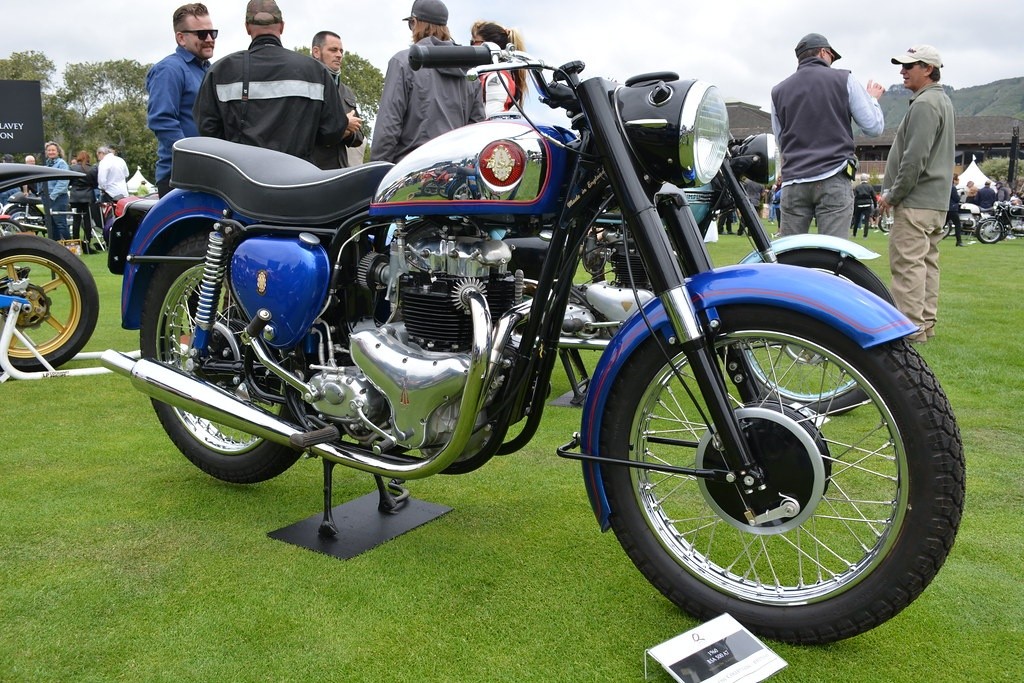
719;232;728;235
1005;234;1016;240
727;231;734;234
956;243;968;247
737;231;744;237
771;232;781;238
748;233;752;238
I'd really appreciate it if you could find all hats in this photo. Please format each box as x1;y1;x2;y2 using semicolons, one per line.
994;181;1003;186
772;184;778;189
966;181;974;189
402;0;448;25
2;154;14;163
794;32;842;65
245;0;283;25
1010;196;1018;202
891;44;944;69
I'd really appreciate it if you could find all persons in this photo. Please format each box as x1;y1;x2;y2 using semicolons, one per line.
878;43;955;343
69;150;92;242
146;3;219;330
737;178;765;236
941;173;968;246
193;0;349;163
312;31;364;169
852;173;878;239
869;202;879;229
345;135;367;166
717;210;735;234
370;0;485;164
470;19;528;121
762;176;781;238
955;181;974;204
92;144;129;229
70;159;77;165
23;155;38;197
770;33;885;241
966;181;1010;208
37;140;71;240
138;181;149;197
0;154;21;208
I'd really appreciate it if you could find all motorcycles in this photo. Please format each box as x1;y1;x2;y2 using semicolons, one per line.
102;41;968;648
0;163;100;370
878;188;1024;244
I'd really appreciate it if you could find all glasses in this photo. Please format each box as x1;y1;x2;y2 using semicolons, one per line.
469;39;485;45
180;29;218;40
902;62;922;70
817;47;836;63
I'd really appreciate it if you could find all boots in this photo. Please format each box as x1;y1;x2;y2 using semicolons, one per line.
81;240;99;254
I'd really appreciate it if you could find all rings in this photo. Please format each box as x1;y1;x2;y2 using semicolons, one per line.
881;205;883;209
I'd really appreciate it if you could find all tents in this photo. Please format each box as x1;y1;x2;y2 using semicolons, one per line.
955;161;998;192
